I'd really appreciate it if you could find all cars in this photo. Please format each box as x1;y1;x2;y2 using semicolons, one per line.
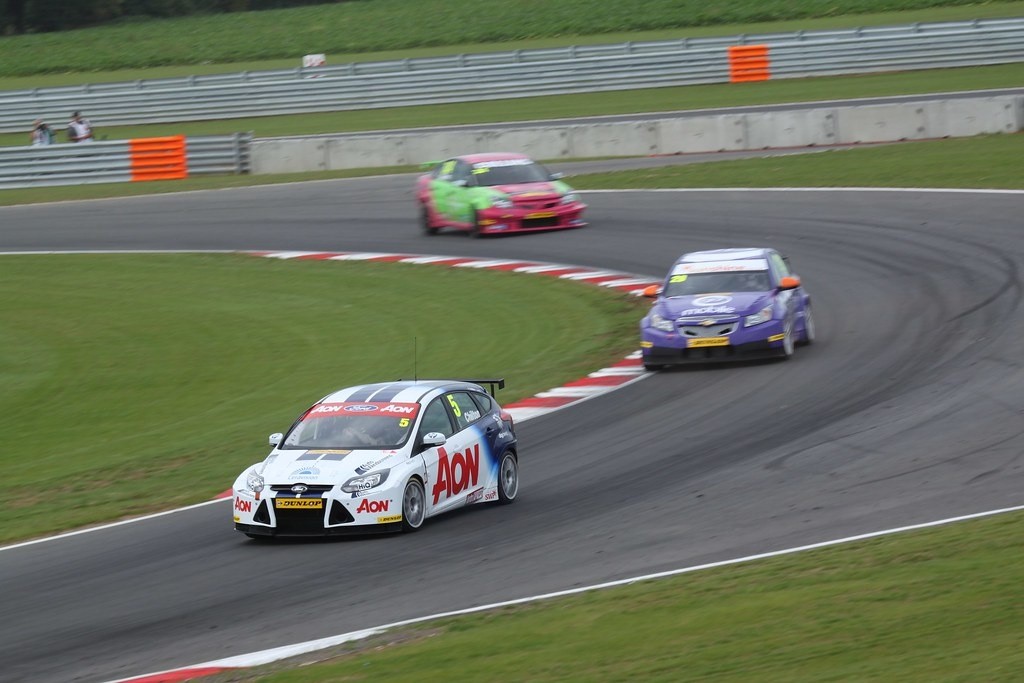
415;150;594;241
639;247;815;371
229;333;521;536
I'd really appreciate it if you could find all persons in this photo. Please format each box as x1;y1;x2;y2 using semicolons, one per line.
28;117;57;146
65;108;95;142
